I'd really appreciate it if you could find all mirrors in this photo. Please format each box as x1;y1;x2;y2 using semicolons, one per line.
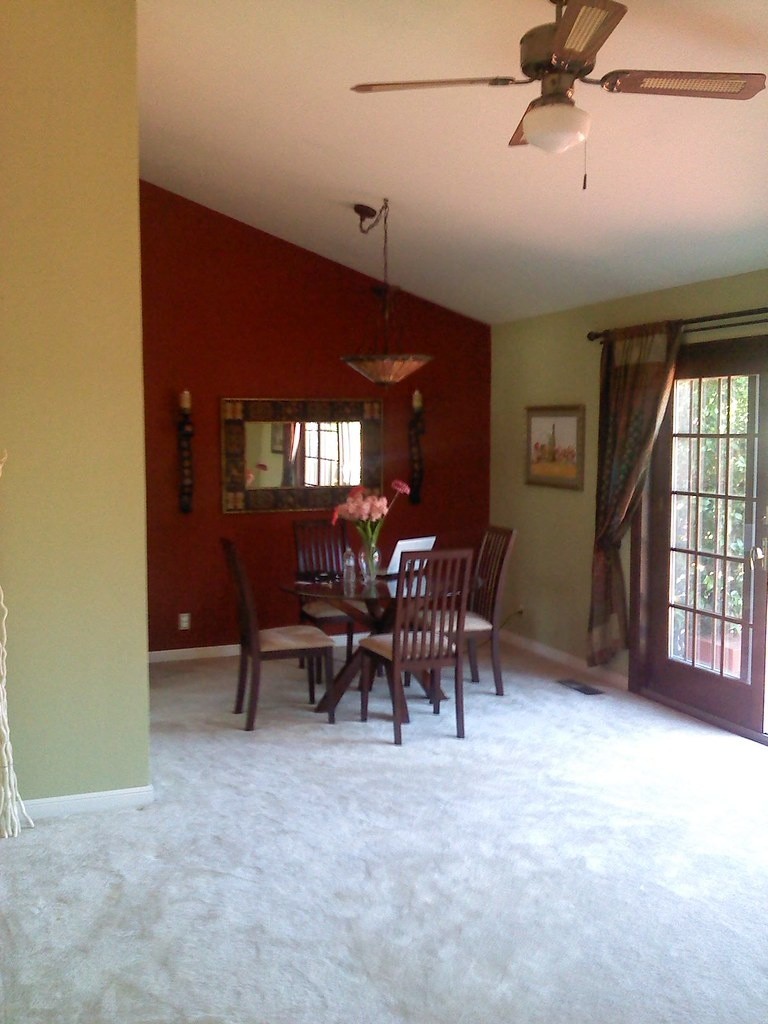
219;397;384;516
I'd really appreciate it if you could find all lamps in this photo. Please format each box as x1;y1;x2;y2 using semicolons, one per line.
337;197;433;387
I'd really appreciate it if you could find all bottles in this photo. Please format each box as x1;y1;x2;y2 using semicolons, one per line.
342;545;356;583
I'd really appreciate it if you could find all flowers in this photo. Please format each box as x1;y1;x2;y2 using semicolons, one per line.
331;480;412;541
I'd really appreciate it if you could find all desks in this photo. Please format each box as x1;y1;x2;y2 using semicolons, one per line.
274;568;486;712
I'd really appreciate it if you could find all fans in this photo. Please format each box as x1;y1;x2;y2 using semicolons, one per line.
350;0;767;191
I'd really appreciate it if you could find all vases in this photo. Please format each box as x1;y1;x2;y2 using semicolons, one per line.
356;542;383;585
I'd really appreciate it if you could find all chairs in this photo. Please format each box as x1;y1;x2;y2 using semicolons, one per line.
290;515;384;676
404;522;518;697
357;547;476;745
222;536;338;731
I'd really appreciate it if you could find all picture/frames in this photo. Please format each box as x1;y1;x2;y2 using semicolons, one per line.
525;403;586;491
